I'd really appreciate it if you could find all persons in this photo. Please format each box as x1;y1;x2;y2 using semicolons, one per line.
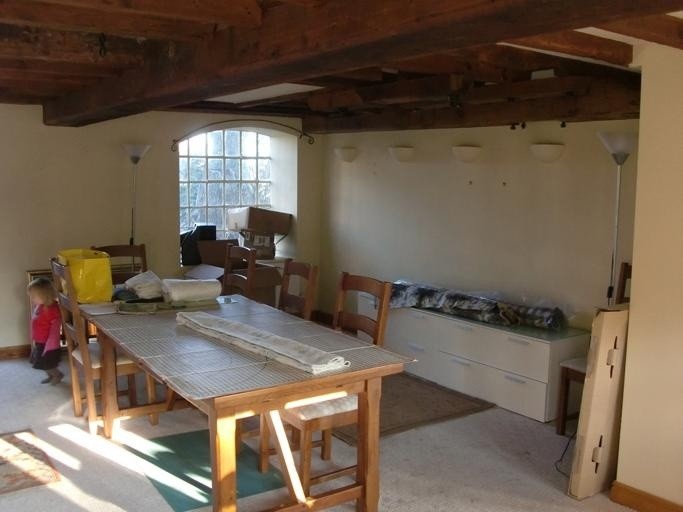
24;278;66;387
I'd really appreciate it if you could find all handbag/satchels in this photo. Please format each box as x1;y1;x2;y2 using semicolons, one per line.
56;249;113;304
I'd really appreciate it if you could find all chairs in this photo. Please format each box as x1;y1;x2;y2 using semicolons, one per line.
556;262;632;435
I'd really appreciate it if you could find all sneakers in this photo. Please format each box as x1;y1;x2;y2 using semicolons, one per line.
41;373;64;385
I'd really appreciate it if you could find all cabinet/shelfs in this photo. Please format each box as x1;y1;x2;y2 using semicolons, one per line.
357;294;590;424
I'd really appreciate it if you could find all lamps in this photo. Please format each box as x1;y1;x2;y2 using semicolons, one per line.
121;143;150;244
596;131;638;306
